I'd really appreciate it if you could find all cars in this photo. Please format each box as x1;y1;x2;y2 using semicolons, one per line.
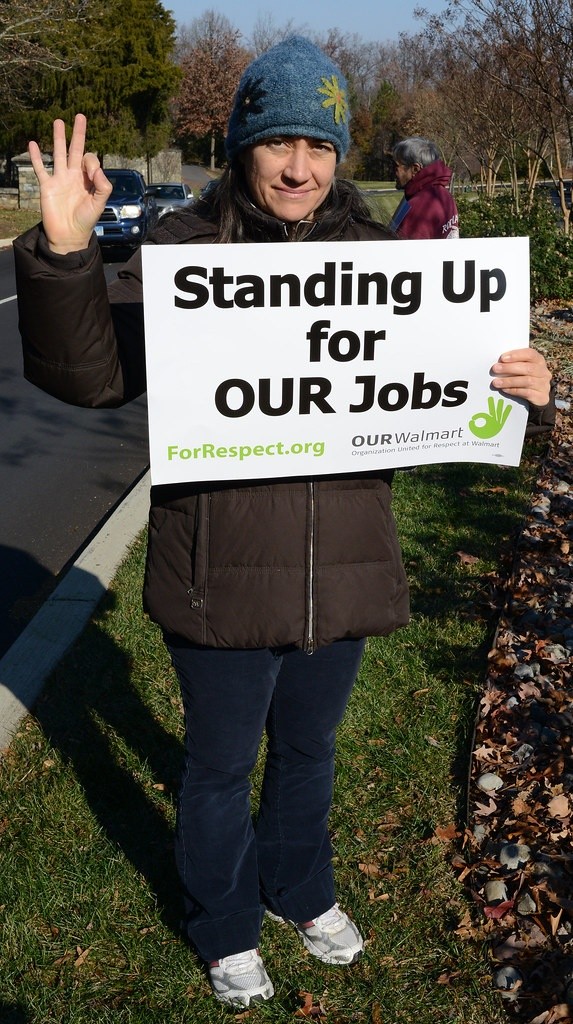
92;168;152;251
141;182;198;219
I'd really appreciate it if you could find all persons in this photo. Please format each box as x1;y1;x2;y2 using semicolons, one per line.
29;35;554;1008
388;138;459;240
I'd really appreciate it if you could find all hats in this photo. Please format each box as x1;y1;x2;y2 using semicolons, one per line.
226;35;349;165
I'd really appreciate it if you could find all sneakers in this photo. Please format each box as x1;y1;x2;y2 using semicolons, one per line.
208;948;274;1008
265;903;363;965
